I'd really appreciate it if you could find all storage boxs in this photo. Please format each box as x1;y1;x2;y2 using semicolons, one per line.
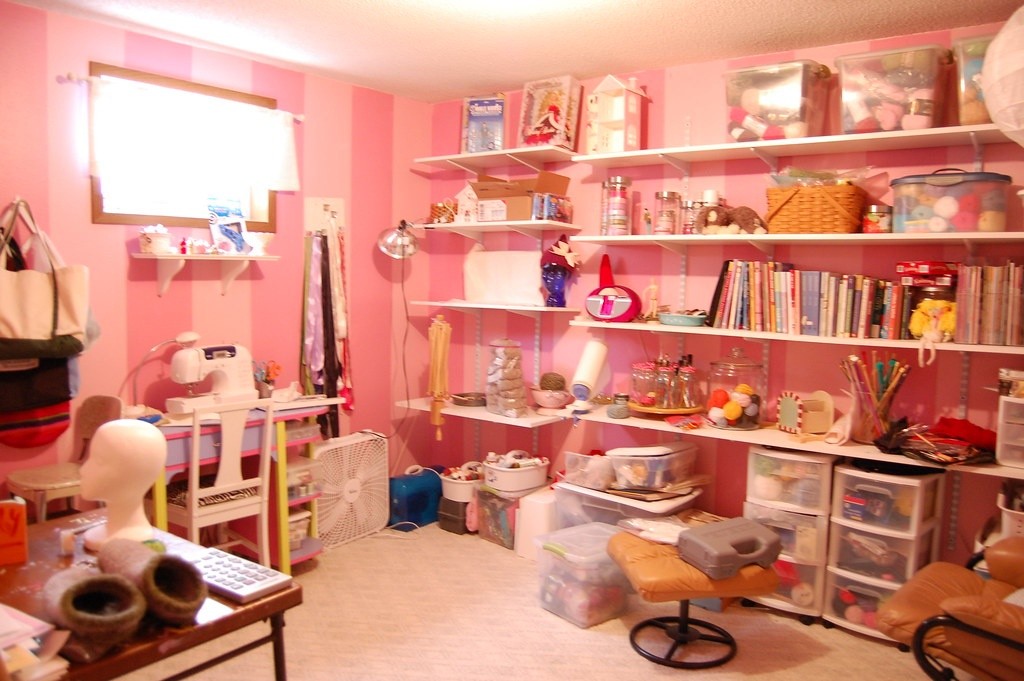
890;168;1013;233
440;455;548;550
536;442;700;629
724;34;999;143
972;490;1024;578
469;170;571;222
995;393;1024;469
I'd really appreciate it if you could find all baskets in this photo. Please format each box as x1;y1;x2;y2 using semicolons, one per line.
763;183;865;235
424;204;458;225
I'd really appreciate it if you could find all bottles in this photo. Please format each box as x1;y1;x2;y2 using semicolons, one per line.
912;287;955;343
704;344;764;431
863;205;892;233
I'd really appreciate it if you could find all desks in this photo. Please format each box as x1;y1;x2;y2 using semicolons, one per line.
0;506;303;681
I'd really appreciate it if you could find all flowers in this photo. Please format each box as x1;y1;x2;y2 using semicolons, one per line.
255;359;281;383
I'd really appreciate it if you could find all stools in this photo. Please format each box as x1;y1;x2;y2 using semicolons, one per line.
606;529;781;670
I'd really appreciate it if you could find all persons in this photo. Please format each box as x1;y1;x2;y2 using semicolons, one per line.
78;418;167;551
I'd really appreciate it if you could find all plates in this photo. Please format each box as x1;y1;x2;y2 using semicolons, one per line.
658;310;706;326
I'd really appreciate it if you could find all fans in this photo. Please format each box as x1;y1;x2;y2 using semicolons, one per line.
307;429;389;552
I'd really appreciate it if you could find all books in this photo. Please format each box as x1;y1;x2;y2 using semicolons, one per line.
707;257;912;340
953;260;1024;348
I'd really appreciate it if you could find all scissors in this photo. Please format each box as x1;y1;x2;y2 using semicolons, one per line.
266;360;282;381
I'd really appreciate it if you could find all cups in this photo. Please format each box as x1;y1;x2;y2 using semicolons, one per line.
850;383;898;443
652;189;719;236
630;362;697;408
601;175;632;234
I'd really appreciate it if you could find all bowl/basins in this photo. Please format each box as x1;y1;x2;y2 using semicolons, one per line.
138;232;174;253
530;385;570;409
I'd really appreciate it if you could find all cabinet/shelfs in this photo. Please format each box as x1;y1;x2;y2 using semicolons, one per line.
568;123;1024;480
414;144;578;428
228;419;324;566
822;462;945;653
742;445;841;625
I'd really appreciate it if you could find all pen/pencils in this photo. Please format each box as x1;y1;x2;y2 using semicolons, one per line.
839;350;911;442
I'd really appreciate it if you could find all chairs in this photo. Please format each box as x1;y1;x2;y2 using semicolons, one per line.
6;396;122;525
875;536;1024;681
143;398;276;568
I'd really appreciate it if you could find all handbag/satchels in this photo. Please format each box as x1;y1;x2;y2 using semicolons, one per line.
0;334;83;411
1;196;89;342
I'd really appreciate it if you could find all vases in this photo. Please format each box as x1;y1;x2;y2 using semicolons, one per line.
257;381;275;398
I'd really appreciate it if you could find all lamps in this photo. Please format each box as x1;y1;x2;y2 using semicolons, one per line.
124;331;200;418
377;219;421;259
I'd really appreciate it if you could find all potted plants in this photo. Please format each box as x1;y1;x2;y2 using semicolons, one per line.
530;372;570;408
